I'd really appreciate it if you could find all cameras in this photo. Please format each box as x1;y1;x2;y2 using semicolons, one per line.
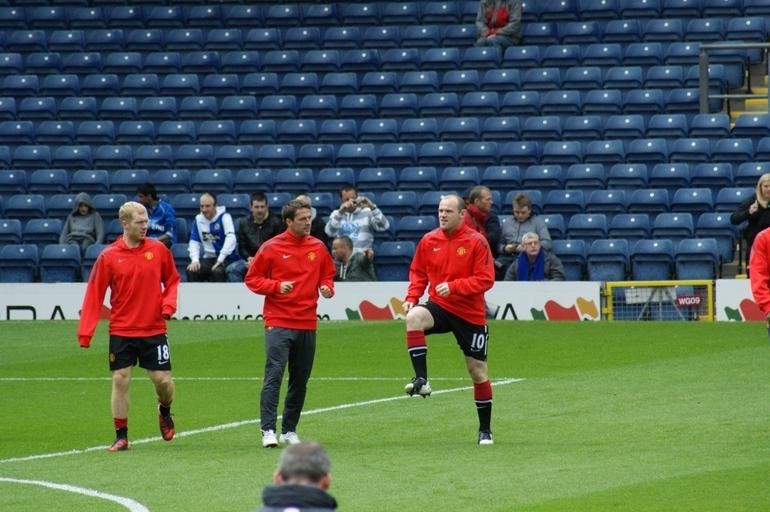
352;199;361;206
494;260;504;273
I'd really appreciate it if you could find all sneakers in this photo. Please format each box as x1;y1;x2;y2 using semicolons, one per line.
405;377;432;398
262;429;278;447
280;432;299;445
109;437;129;451
157;405;174;441
478;430;494;445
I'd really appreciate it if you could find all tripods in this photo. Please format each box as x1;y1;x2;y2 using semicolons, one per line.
634;286;686;321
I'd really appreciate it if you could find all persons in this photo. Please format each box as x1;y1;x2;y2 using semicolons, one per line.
474;0;525;47
403;191;497;446
136;183;176;254
461;185;498;263
58;191;106;256
184;190;241;282
495;193;554;280
244;200;336;449
78;201;183;451
458;190;504;255
224;190;286;282
341;236;375;262
294;194;335;259
328;234;381;281
253;438;340;512
325;183;391;265
725;172;769;275
502;232;567;281
747;225;770;332
